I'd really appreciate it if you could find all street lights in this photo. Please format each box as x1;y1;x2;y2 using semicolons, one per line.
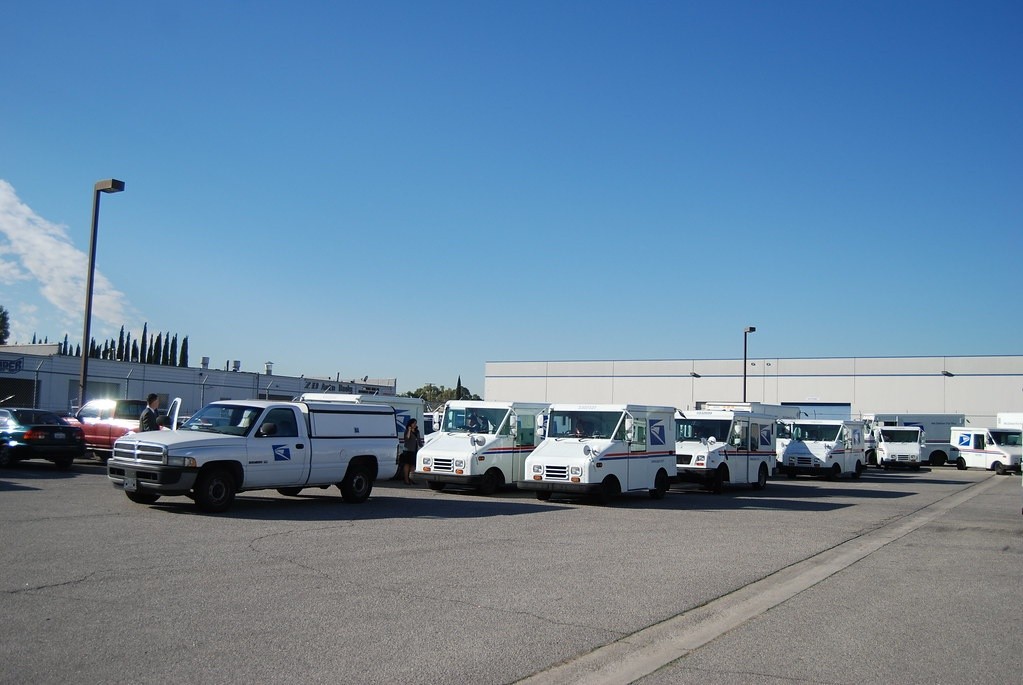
78;179;125;407
743;327;756;402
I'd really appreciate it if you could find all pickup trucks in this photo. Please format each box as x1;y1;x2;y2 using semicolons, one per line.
61;397;159;462
106;396;401;513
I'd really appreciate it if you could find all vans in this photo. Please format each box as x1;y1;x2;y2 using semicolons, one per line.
949;426;1023;475
782;419;866;480
674;410;778;492
517;404;677;505
877;426;925;471
408;400;568;495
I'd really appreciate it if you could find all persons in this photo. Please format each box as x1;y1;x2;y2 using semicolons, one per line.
140;393;160;432
467;414;487;432
816;430;823;440
883;433;892;442
576;420;587;438
403;418;423;484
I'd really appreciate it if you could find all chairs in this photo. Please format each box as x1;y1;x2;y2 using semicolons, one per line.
694;427;708;440
583;422;593;436
607;420;625;439
477;417;488;434
237;409;255;427
807;430;817;440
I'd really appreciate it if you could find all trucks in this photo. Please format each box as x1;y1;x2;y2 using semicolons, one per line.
423;413;466;434
701;402;800;420
293;394;424;460
863;414;965;466
997;412;1023;430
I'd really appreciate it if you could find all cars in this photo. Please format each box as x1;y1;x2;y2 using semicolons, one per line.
0;408;87;470
176;417;213;429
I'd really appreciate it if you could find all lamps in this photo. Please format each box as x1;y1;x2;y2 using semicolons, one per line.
941;371;948;375
690;372;696;375
